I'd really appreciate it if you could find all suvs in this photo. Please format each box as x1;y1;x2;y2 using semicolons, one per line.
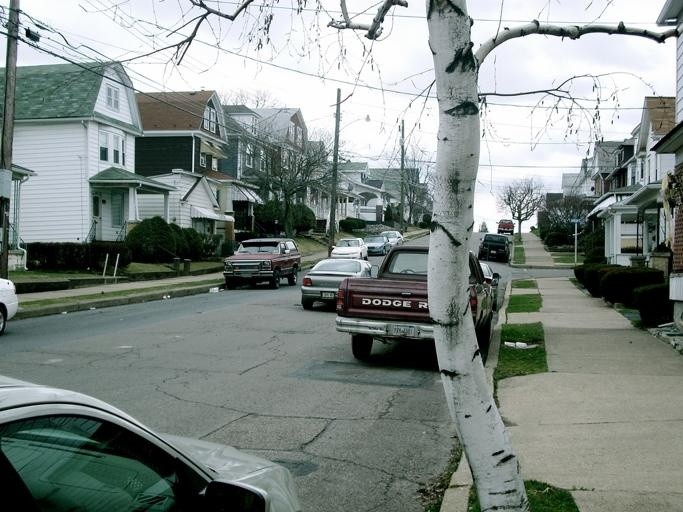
477;233;514;265
496;219;517;236
223;236;302;291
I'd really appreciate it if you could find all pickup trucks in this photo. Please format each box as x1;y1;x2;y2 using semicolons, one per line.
334;243;497;366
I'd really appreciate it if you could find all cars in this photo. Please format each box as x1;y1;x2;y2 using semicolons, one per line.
300;257;374;311
0;372;303;511
479;261;502;311
381;231;406;249
362;234;392;258
0;276;20;337
330;237;370;262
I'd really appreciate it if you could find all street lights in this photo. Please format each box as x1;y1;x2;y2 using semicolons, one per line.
327;88;373;258
400;118;426;236
